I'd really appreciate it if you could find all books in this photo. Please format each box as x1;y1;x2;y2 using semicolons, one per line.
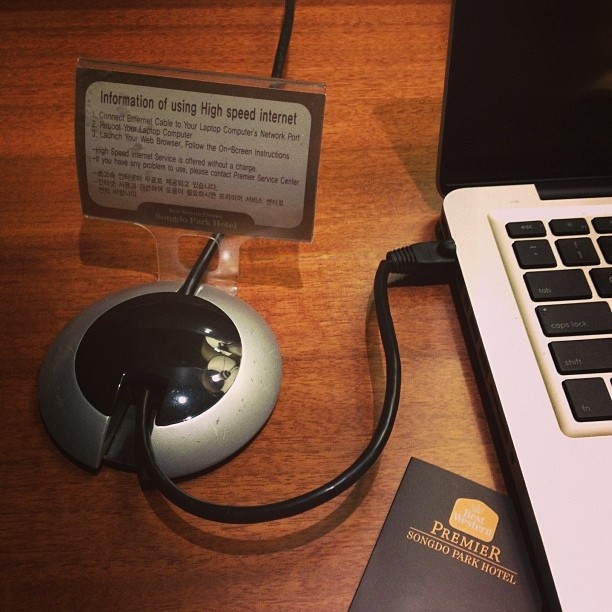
349;457;561;612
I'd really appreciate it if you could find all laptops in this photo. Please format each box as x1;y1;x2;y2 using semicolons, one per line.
435;3;612;612
